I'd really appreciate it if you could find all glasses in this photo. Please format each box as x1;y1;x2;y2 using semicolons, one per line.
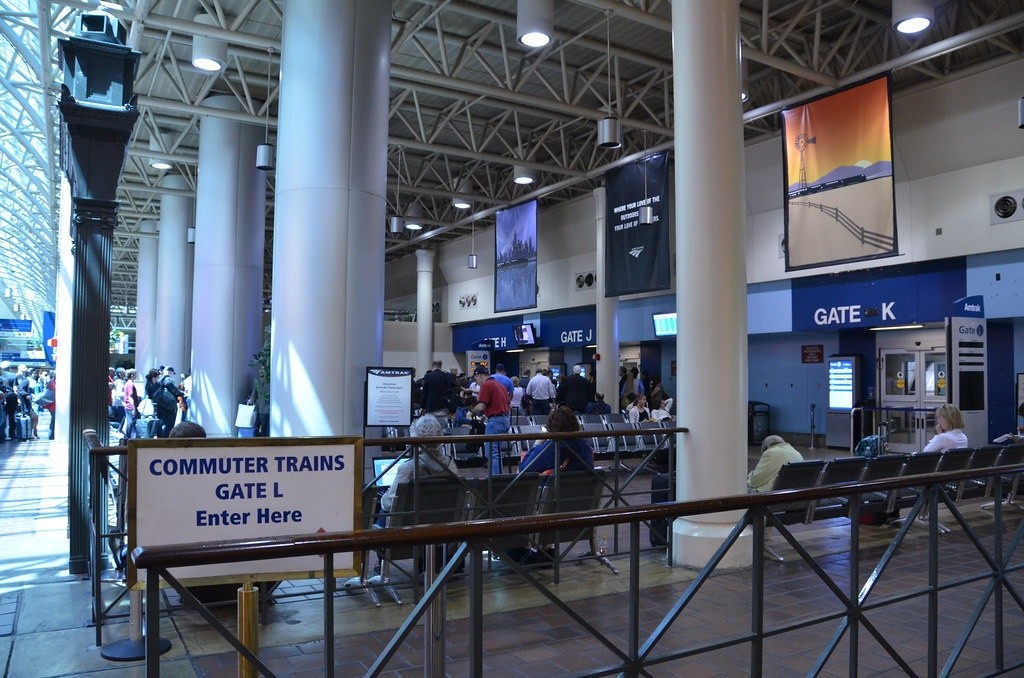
942;404;946;412
31;373;37;374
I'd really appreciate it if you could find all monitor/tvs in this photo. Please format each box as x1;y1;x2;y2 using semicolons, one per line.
511;323;539;349
829;359;852;409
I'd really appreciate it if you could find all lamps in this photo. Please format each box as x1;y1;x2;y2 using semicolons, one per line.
596;7;627;152
148;127;176;170
5;288;14;298
511;146;538;185
450;174;472;209
255;46;274;171
467;200;478;269
637;127;656;226
890;0;938;39
514;0;554;49
191;11;229;74
405;200;425;230
20;313;27;320
1017;96;1024;130
390;144;403;234
187;163;199;244
742;57;752;102
14;303;20;311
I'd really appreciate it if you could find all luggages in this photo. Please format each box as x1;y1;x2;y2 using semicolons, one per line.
856;421;889;457
15;397;34;440
135;414;160;439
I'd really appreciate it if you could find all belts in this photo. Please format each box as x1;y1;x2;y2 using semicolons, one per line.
488;414;508;418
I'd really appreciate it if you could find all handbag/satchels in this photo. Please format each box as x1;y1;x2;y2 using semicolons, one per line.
33;384;55;408
118;416;127;435
234;400;257;427
154;387;176;411
464;413;488;454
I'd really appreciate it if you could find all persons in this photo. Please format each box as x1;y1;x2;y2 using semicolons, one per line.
248;366;270;437
619;366;676;423
1006;402;1024;443
585;391;611;414
0;361;56;441
167;422;226;602
411;358;488;451
471;366;511;475
373;416;458;574
748;436;803;492
490;364;594;415
109;366;192;439
915;405;968;524
492;407;592;564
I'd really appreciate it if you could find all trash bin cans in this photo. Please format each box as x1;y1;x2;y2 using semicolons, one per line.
748;401;770;445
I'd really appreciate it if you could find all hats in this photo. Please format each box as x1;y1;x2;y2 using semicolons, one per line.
42;372;48;375
495;364;504;371
18;364;27;371
761;436;786;451
472;366;489;374
573;365;581;374
1;361;10;369
164;367;176;374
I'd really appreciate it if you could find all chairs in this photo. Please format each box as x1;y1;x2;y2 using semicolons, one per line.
355;408;1024;605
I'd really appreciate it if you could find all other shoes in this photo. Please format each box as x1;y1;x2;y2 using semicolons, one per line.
525;548;556;564
374;563;381;574
